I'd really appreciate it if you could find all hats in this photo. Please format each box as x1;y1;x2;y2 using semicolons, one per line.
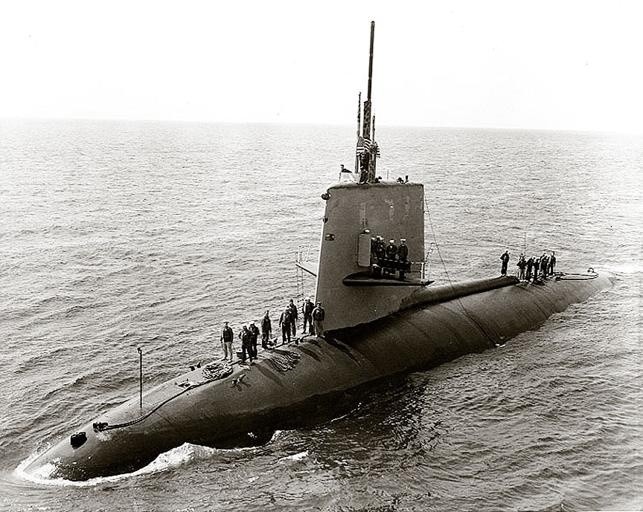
371;238;375;241
380;238;384;241
377;236;381;238
400;239;406;242
389;240;394;243
365;230;370;233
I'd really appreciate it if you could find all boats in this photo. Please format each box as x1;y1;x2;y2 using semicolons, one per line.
26;20;613;483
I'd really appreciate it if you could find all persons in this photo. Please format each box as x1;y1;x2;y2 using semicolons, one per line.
287;299;298;336
371;235;408;262
219;321;234;363
239;326;253;364
311;302;325;339
301;299;314;334
278;309;293;344
500;251;509;277
517;251;556;280
339;164;351;180
248;321;259;359
260;310;272;348
